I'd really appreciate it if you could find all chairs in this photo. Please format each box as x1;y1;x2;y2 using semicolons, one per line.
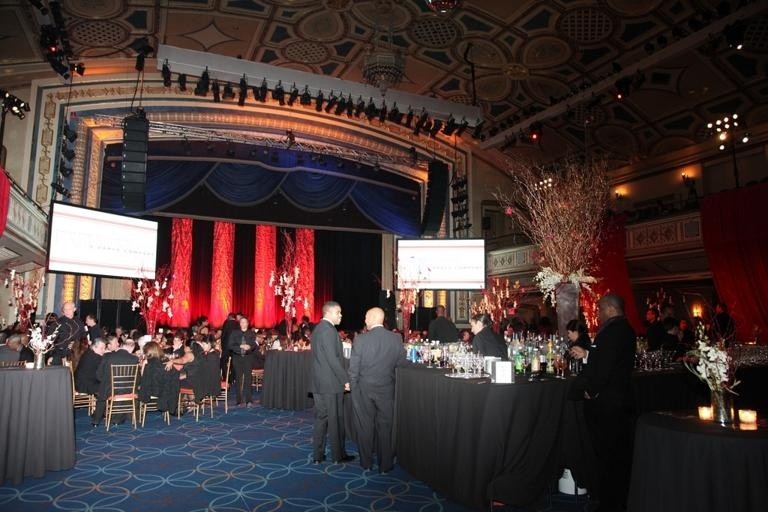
105;363;138;432
140;367;170;430
214;355;232;415
64;361;94;416
178;364;214;423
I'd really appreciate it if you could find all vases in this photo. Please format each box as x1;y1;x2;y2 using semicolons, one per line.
554;281;580;343
32;350;46;369
710;378;733;422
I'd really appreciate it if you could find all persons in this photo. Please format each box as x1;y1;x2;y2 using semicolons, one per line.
140;342;163;377
500;318;513;342
713;304;736;346
228;317;255;409
164;333;184;359
197;316;208;336
569;291;635;443
115;327;123;337
106;335;120;352
12;322;20;332
291;317;300;341
306;301;355;463
347;306;400;474
676;319;694;344
90;339;142;428
459;330;470;342
74;337;107;396
253;331;266;369
661;317;683;359
47;302;85;365
555;320;591;369
6;335;35;363
302;327;311;343
160;334;173;353
222;313;244;382
162;338;203;413
70;335;88;371
200;334;216;355
0;331;20;361
86;313;106;343
155;328;167;347
647;304;674;351
427;305;458;344
302;316;312;327
223;312;235;340
471;316;507;362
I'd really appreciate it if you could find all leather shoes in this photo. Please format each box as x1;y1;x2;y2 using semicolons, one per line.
333;456;355;464
111;423;117;429
314;455;326;464
246;401;252;408
380;465;393;474
237;403;241;407
364;467;371;471
91;423;98;428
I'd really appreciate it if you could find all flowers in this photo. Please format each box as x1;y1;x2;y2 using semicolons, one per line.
487;151;607;305
682;322;731;388
22;310;79;354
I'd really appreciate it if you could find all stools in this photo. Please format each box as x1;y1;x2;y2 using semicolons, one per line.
252;369;264;392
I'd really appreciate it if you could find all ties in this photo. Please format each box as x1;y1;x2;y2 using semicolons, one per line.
240;335;247;357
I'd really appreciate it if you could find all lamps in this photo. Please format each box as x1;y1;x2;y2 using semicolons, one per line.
51;183;72;198
59;146;76;161
259;77;267;103
277;80;285;105
287;83;299;105
355;96;363;120
455;121;469;136
353;95;365;119
324;91;338;113
135;52;144;72
379;100;387;123
163;64;170;86
316;89;323;111
60;125;77;142
57;164;71;177
336;92;345;117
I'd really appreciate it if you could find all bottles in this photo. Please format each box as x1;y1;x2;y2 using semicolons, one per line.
507;332;583;378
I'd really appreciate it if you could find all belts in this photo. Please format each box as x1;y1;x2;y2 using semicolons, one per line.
234;352;248;356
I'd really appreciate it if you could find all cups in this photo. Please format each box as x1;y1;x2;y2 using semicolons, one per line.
450;354;485;376
553;354;564;378
558;358;567;379
